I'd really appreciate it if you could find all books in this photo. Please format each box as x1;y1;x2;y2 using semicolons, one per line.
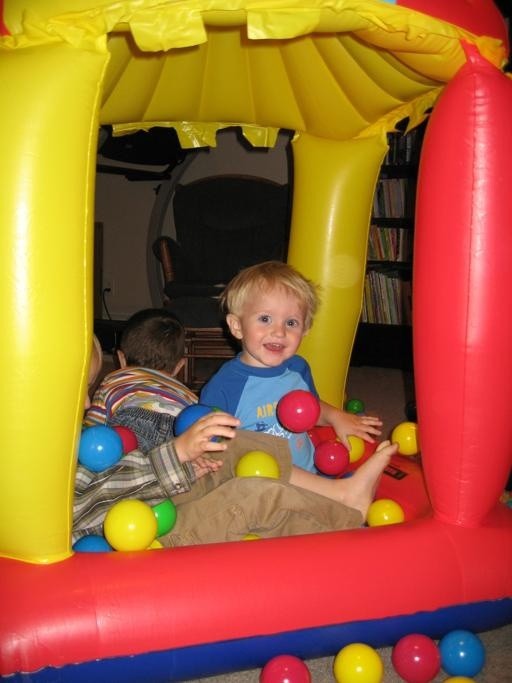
359;126;417;326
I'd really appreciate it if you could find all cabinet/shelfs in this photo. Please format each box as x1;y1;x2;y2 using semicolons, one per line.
354;115;430;368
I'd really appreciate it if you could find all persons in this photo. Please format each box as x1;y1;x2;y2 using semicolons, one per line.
81;307;199;456
198;258;385;475
73;335;400;552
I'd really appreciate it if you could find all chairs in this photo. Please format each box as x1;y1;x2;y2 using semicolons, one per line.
150;174;289;387
